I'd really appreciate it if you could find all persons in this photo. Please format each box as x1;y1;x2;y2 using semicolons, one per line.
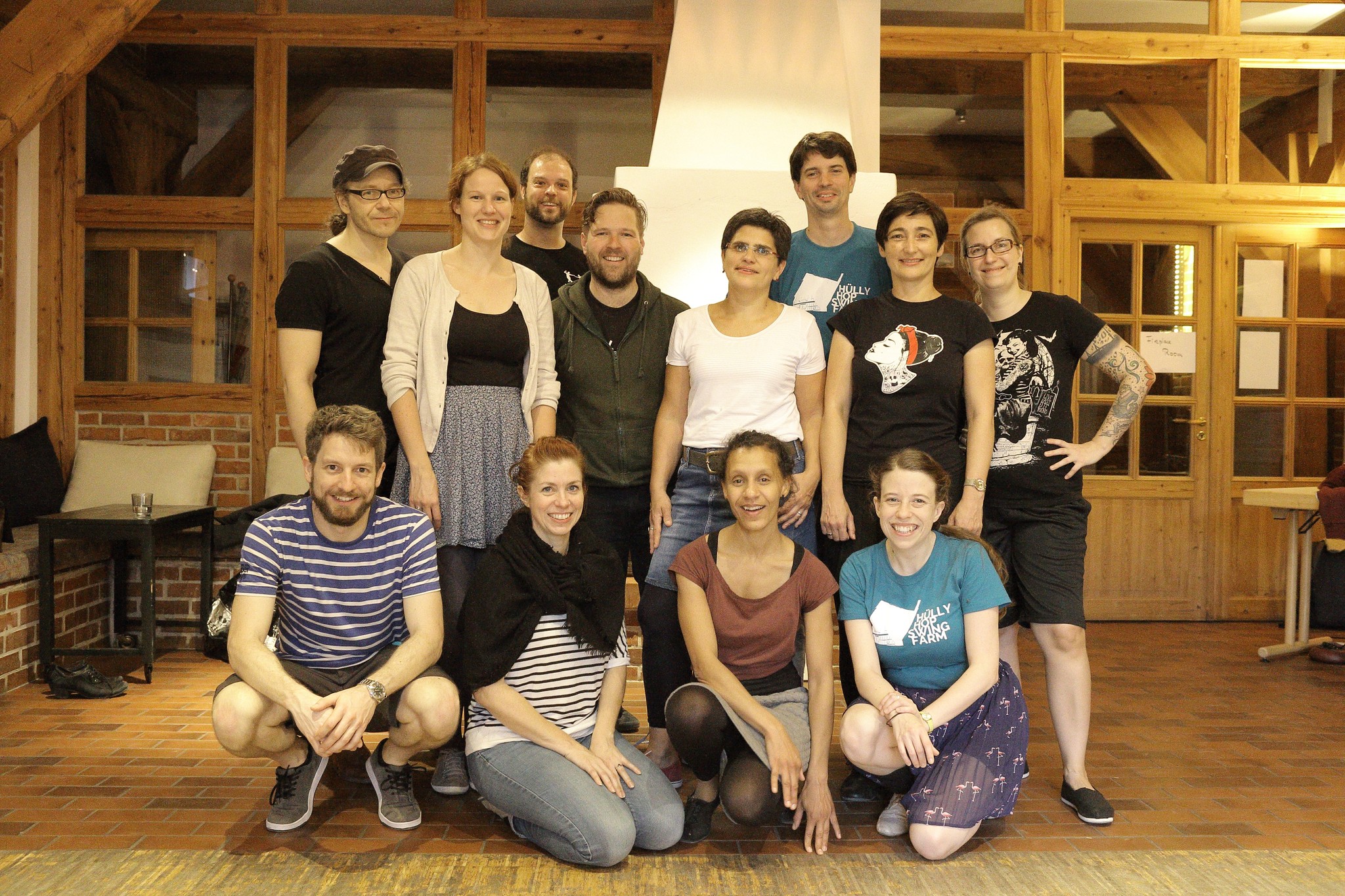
273;129;1000;780
213;406;461;831
452;435;684;866
832;446;1026;865
666;432;843;856
960;210;1156;824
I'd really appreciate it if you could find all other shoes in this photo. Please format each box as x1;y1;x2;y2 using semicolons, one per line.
1060;775;1115;823
478;796;508;818
615;705;639;732
1022;760;1029;779
331;732;372;783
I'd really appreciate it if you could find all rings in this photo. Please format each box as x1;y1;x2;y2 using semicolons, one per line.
896;694;900;700
798;511;802;515
827;531;832;535
615;764;623;769
648;526;654;532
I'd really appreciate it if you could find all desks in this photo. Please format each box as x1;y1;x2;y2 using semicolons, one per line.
1242;487;1332;657
37;503;216;684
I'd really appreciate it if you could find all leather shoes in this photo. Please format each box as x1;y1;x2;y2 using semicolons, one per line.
839;761;883;802
679;790;720;844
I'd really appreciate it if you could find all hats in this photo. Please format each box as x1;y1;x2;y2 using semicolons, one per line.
333;145;403;188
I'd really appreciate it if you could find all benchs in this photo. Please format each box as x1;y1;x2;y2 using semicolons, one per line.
112;522;249;562
0;523;113;584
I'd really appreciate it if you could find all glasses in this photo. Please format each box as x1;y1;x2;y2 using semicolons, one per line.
725;242;780;258
344;186;405;200
964;239;1018;258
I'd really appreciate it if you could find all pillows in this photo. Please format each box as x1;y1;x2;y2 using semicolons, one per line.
261;446;308;494
0;417;64;526
61;440;217;512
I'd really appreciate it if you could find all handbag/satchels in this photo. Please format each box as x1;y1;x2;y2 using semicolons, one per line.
202;572;280;663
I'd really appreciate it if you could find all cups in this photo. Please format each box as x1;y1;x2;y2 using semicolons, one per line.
131;493;153;518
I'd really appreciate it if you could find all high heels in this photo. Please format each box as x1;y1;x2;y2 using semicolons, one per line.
46;660;128;697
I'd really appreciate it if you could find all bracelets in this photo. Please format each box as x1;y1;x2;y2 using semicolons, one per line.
886;712;902;727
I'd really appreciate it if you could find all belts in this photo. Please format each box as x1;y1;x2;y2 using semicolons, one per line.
682;440;802;475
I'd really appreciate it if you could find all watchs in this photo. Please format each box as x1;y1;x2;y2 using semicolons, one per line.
964;478;986;493
357;678;387;706
919;711;933;734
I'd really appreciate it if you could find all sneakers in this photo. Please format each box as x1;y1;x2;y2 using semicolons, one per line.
365;737;426;830
644;758;683;787
266;744;329;832
431;747;469;794
876;793;911;836
